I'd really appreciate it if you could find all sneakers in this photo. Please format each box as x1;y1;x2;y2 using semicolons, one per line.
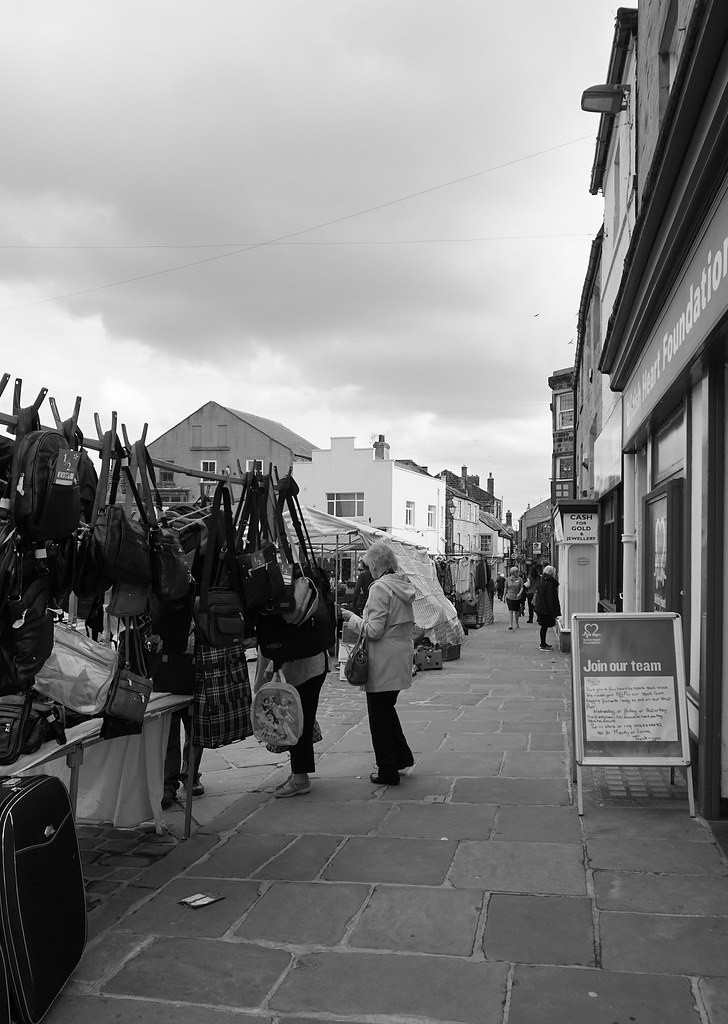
183;778;204;796
161;783;176;809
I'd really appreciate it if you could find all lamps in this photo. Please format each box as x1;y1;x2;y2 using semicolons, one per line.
580;83;630;114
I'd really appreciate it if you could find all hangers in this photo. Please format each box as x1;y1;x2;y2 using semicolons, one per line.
429;552;482;563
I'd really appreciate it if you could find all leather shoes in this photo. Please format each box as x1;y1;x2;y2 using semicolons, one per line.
370;772;380;783
397;756;414;770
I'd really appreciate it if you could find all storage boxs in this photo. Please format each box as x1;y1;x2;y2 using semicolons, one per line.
414;649;442;668
440;642;461;661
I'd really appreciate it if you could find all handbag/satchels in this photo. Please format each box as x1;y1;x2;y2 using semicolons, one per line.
344;622;369;686
531;590;538;606
0;373;337;754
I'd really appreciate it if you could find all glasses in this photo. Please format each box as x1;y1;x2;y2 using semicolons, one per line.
516;571;518;573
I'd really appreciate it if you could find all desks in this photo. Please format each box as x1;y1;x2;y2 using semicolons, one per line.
0;694;193;840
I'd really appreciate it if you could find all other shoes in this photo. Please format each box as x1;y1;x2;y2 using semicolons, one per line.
508;626;512;630
273;774;311;789
273;779;312;797
516;625;519;628
527;621;533;623
518;613;521;617
522;611;524;616
540;644;553;651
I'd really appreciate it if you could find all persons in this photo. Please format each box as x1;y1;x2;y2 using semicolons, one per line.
341;543;416;785
253;551;331;797
353;562;375;614
494;565;542;629
534;565;562;652
146;620;205;809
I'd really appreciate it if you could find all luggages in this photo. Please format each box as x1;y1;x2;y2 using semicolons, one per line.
0;774;87;1024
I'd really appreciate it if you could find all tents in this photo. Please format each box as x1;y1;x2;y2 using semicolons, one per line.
236;506;465;663
